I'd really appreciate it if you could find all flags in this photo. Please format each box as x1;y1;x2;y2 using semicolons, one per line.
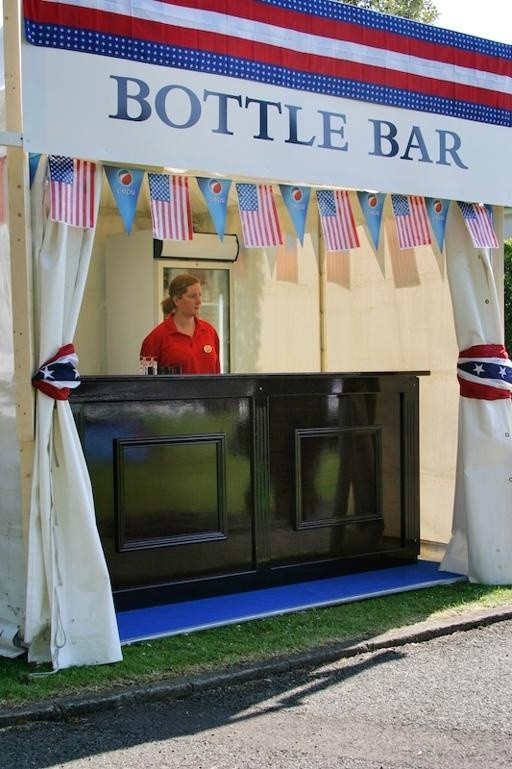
454;201;500;250
47;156;98;231
391;194;433;251
315;189;360;253
146;173;195;241
232;181;284;249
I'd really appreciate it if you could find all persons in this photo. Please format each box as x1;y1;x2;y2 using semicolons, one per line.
135;273;222;374
